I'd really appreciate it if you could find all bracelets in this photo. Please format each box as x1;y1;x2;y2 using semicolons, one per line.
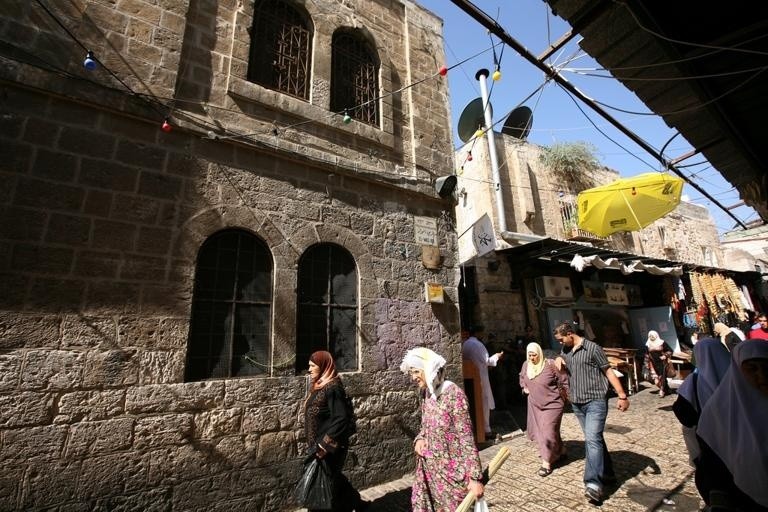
619;396;627;401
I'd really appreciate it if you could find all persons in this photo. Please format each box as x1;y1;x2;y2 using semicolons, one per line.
551;320;630;505
695;338;768;511
304;349;371;511
713;323;748;352
516;341;568;477
399;347;486;512
461;324;505;438
672;337;732;470
643;328;674;399
746;311;768;339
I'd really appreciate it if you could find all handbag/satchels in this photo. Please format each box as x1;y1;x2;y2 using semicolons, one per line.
551;367;566;400
667;362;676;378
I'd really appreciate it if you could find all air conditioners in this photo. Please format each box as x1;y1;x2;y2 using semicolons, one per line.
534;275;575;304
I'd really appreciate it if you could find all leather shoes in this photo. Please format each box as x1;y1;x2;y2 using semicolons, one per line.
585;483;602;502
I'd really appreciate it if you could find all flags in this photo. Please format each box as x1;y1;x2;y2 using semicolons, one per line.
471;214;498;259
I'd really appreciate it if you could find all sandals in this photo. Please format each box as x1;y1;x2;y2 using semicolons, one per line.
538;466;552;476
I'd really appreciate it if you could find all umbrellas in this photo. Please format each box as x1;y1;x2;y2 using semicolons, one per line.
575;169;685;240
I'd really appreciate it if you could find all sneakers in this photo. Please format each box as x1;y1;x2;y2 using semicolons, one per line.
485;431;501;439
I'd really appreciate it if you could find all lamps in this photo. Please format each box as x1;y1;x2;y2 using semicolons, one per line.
435;174;457;198
